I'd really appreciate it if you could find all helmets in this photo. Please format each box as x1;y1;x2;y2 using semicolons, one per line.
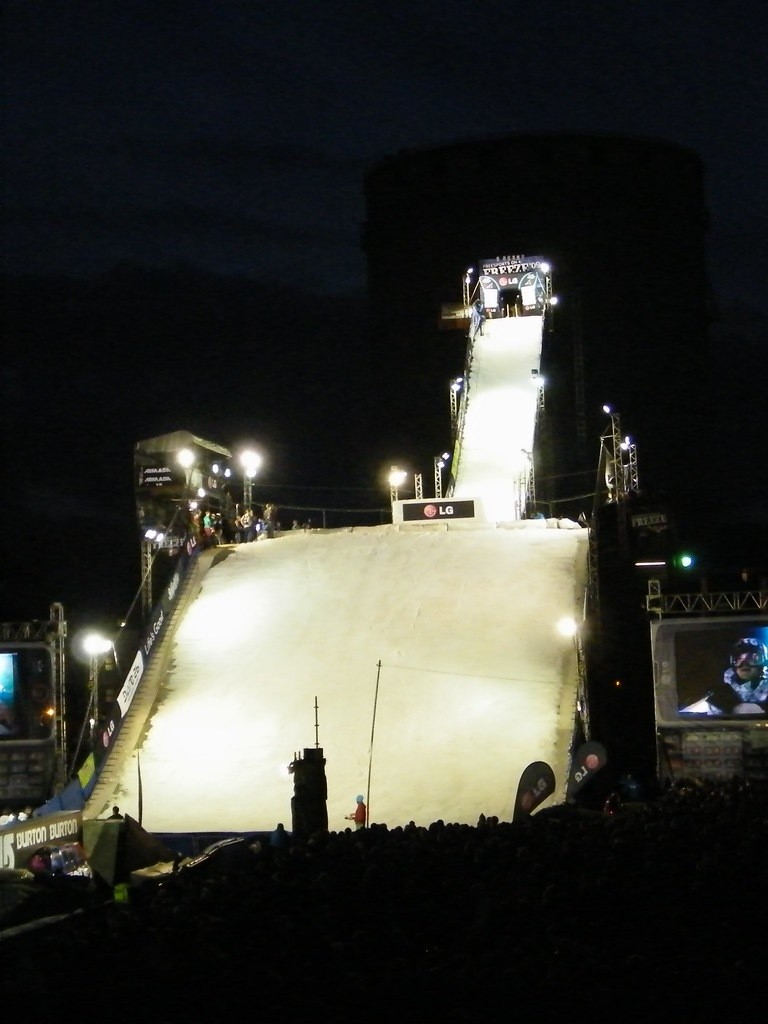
729;636;768;667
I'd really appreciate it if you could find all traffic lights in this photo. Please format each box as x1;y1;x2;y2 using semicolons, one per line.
669;554;696;568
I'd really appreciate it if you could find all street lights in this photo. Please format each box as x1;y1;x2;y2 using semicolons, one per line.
560;617;593;743
388;465;401;510
240;448;255;518
83;633;110;744
434;453;449;499
449;376;464;459
603;405;624;503
620;435;639;497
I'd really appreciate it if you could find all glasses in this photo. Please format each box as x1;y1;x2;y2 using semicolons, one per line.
730;651;766;666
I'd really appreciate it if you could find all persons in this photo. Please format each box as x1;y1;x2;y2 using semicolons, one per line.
348;795;366;830
198;504;277;544
107;806;123;819
1;774;768;1024
292;520;300;529
500;298;506;317
515;295;519;309
723;637;768;707
472;300;485;336
